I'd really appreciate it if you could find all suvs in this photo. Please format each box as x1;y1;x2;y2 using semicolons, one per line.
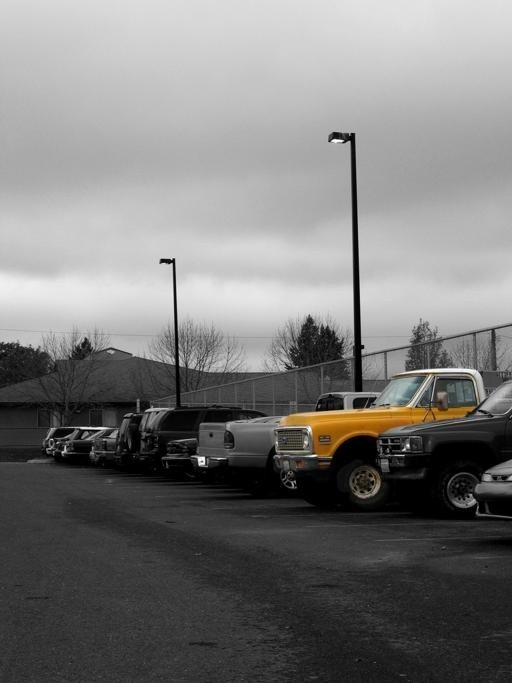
375;379;511;518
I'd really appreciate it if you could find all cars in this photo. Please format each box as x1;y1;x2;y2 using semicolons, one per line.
473;459;512;522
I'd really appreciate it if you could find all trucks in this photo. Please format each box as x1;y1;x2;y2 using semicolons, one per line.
272;365;510;509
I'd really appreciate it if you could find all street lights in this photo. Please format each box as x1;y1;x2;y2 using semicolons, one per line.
157;256;184;410
325;132;365;391
